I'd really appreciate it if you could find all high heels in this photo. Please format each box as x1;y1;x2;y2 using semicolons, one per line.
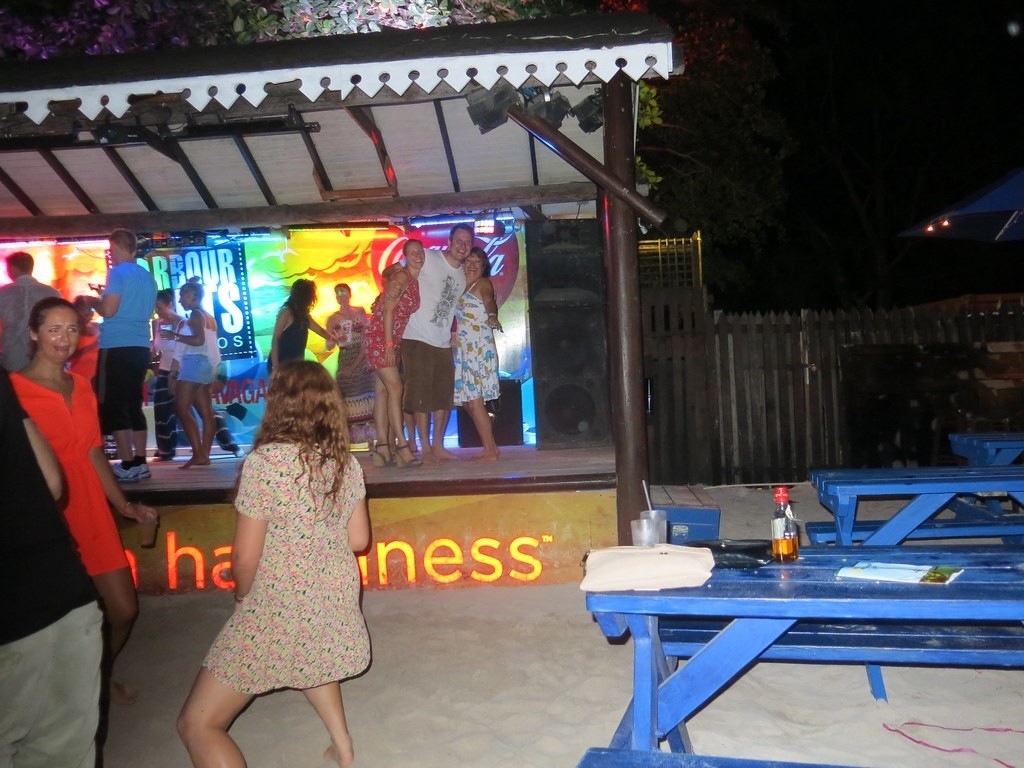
395;444;423;468
371;443;395;467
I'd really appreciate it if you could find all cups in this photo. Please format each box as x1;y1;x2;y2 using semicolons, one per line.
90;298;99;311
337;335;344;346
631;519;660;546
161;325;172;340
137;516;156;545
641;511;666;543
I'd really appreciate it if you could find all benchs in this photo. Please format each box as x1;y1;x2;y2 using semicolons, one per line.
655;615;1023;672
646;484;720;542
806;514;1024;543
574;747;869;768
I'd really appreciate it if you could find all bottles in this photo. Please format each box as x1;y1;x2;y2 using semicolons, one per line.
771;488;799;564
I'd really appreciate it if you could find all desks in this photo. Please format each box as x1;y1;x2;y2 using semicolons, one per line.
808;466;1024;702
949;432;1024;518
586;542;1024;760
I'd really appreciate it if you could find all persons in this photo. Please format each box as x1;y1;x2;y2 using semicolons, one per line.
0;252;100;382
152;276;245;470
175;360;373;768
7;297;159;668
87;230;158;482
267;279;340;380
362;223;504;470
0;363;103;768
326;284;376;456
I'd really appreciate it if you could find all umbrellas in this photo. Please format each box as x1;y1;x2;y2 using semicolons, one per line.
897;168;1024;242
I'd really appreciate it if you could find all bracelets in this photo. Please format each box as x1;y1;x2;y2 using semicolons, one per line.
488;312;497;317
233;591;244;601
384;342;393;346
120;501;131;512
330;335;335;341
174;333;180;341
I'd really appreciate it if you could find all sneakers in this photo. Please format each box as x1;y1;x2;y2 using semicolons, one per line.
112;464;139;482
139;464;151;478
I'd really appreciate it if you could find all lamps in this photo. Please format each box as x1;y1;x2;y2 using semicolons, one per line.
464;79;604;137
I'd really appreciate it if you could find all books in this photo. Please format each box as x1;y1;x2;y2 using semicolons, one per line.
837;560;964;584
